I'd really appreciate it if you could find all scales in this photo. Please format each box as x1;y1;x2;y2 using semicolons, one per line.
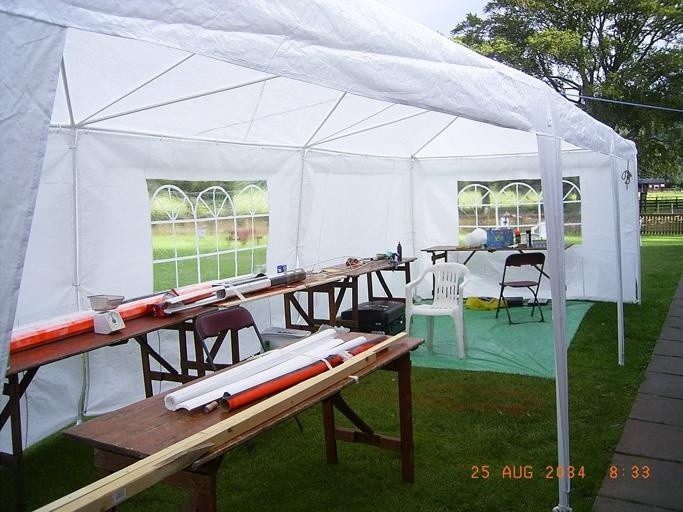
89;294;126;334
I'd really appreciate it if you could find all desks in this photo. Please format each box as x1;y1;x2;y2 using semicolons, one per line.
421;243;573;307
1;307;220;512
59;328;427;510
308;253;421;332
179;272;347;386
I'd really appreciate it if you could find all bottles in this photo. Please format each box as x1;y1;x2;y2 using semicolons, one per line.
515;231;521;243
398;241;401;263
526;229;532;248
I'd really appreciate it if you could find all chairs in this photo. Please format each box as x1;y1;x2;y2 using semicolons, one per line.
494;253;546;325
195;306;305;434
404;262;472;363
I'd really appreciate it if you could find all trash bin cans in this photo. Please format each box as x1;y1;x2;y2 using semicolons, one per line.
341;301;406;371
260;327;311;354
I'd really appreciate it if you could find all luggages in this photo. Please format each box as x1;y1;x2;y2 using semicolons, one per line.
341;301;405;336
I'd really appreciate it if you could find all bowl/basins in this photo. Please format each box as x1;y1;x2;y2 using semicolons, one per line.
375;254;385;261
